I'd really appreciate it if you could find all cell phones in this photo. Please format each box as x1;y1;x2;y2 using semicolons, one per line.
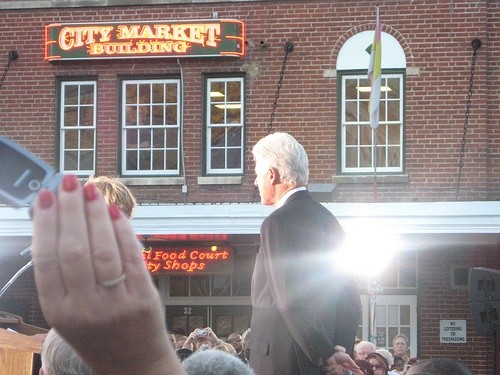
0;136;144;251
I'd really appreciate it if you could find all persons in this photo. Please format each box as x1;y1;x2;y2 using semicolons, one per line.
354;334;473;375
31;176;256;375
248;132;365;375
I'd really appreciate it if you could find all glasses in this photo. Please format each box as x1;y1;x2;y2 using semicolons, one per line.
229;338;243;343
197;337;212;342
357;352;371;356
371;363;386;371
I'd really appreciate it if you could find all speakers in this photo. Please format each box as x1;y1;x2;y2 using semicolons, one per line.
468;266;500;337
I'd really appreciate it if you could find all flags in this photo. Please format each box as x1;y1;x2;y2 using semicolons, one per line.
367;4;381;130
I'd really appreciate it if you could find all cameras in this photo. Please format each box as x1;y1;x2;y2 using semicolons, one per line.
197;329;208;336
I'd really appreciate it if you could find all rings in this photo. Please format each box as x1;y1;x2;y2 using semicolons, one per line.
102;273;127;288
